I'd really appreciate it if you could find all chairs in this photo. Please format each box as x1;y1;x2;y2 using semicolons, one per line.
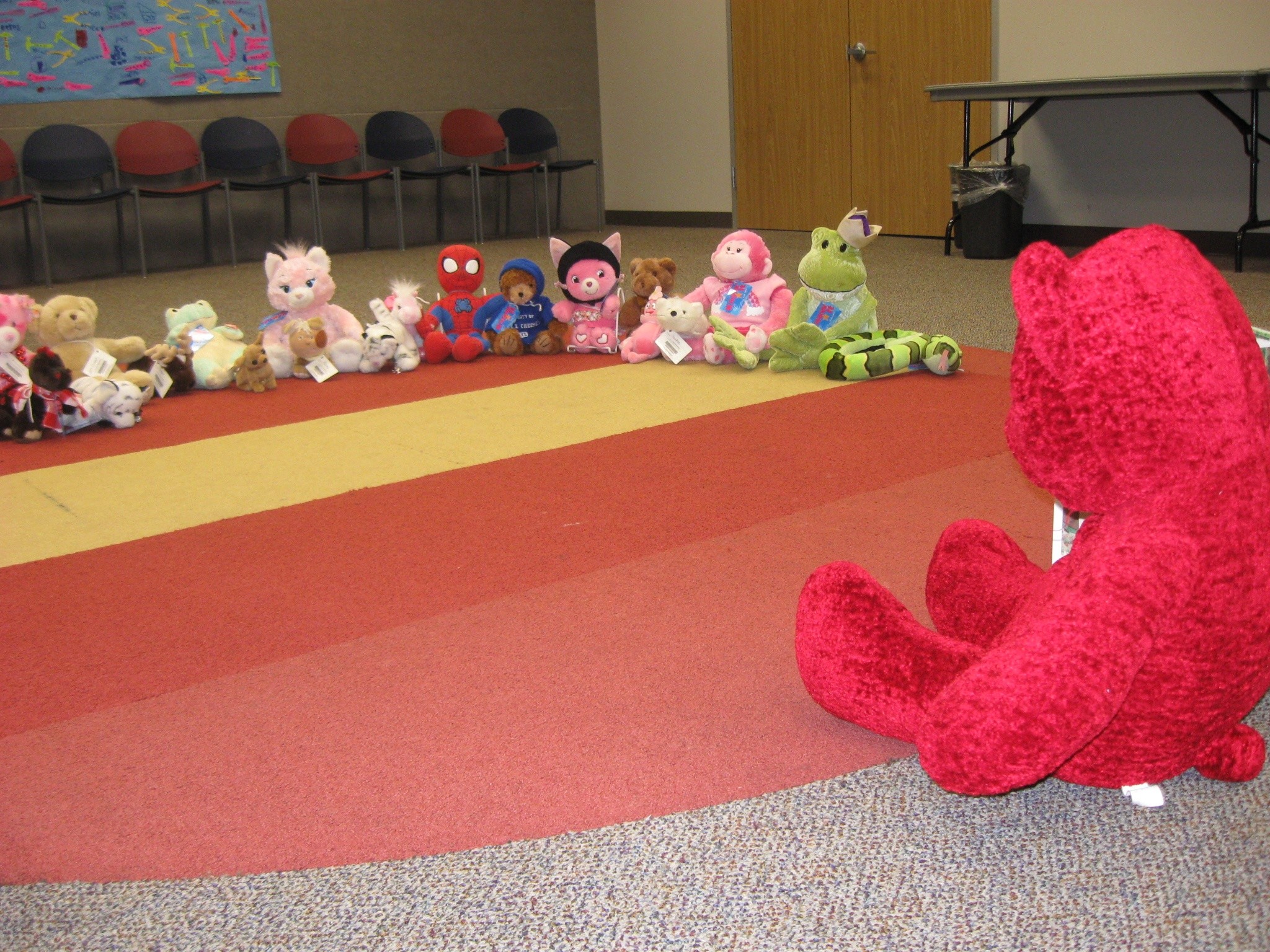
196;112;321;270
436;106;552;245
281;111;406;255
112;118;239;278
363;109;482;253
0;136;51;287
496;106;603;238
16;123;148;284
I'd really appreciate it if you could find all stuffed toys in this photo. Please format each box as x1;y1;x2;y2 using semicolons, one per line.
415;244;505;364
550;233;625;354
0;293;40;441
165;299;247;390
709;206;883;373
117;326;196;398
358;276;430;374
818;329;963;381
3;345;89;443
234;331;277;393
259;237;365;379
23;294;154;405
619;229;794;370
796;224;1270;797
481;258;568;356
61;376;152;435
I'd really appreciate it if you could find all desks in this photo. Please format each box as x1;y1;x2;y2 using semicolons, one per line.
926;66;1270;274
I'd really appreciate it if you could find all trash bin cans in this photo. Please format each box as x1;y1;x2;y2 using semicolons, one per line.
948;163;1031;262
